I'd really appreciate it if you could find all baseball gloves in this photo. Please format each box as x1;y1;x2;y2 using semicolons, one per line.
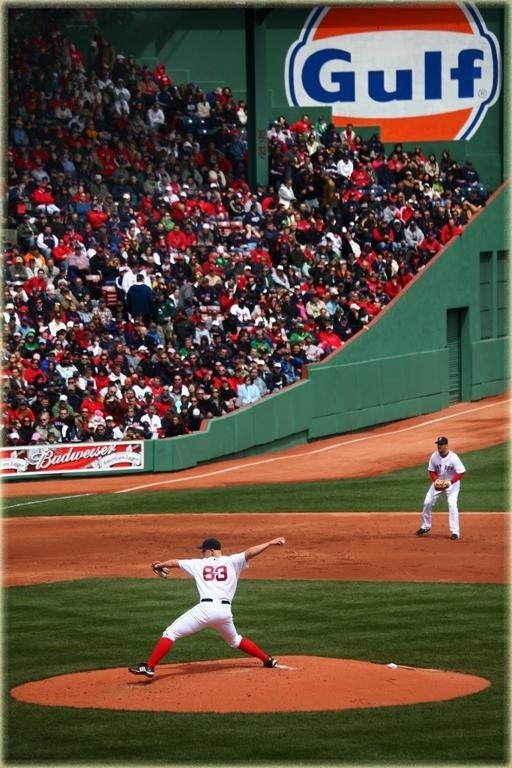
433;479;452;491
152;560;169;580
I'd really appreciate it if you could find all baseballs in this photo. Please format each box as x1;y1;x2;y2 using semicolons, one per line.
278;537;285;546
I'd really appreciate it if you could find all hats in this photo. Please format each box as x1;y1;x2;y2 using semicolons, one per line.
434;436;448;444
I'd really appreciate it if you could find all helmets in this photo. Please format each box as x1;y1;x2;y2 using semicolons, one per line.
196;537;221;550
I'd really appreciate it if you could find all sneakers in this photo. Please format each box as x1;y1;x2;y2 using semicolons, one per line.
264;655;278;667
128;663;155;678
416;527;430;535
450;534;457;540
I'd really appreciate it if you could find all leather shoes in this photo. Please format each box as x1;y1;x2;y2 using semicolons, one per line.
200;597;230;604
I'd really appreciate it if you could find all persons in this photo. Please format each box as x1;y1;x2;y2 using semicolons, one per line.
0;10;489;443
415;436;466;540
128;537;285;678
